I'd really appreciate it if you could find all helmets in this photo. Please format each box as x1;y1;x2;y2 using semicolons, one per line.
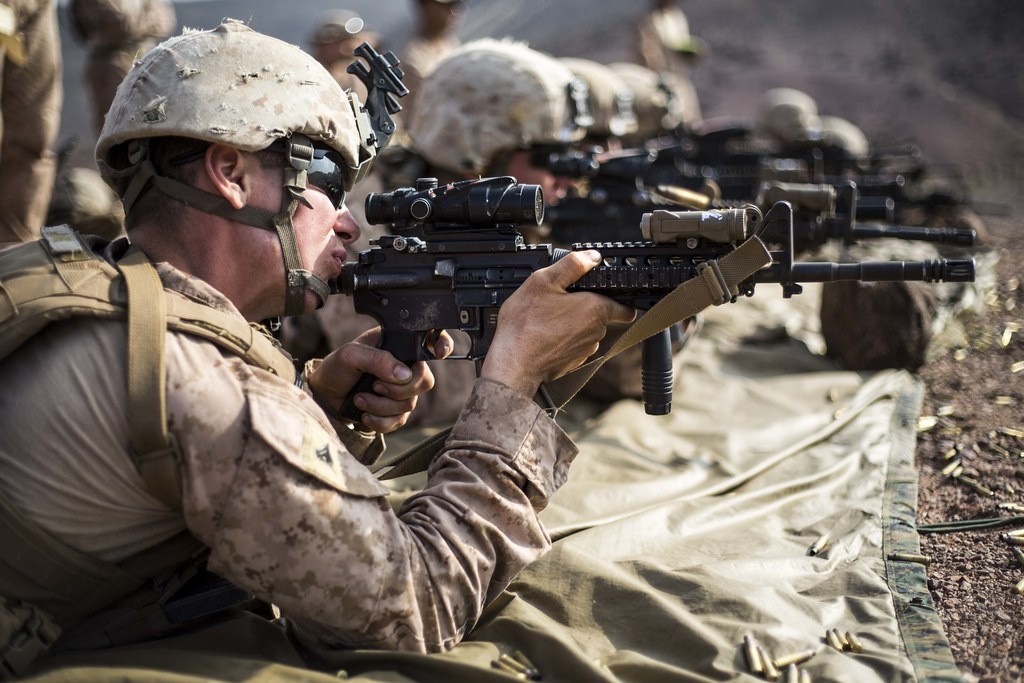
758;90;869;169
406;39;702;175
94;17;376;192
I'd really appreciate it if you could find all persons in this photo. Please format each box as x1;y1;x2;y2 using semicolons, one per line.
2;0;867;429
0;17;640;682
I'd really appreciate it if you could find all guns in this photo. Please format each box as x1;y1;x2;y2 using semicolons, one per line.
325;119;1012;422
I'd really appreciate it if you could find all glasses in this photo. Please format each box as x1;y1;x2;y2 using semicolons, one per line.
307;142;345;208
